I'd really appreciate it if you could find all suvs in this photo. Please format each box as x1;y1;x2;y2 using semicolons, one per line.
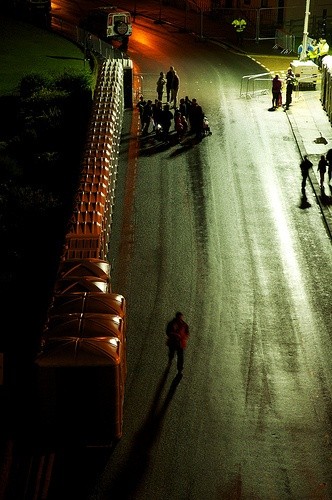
89;6;132;39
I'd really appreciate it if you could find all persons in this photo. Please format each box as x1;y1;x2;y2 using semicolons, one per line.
272;67;295;110
165;312;190;375
301;149;332;186
232;16;247;42
298;38;329;67
136;66;212;137
